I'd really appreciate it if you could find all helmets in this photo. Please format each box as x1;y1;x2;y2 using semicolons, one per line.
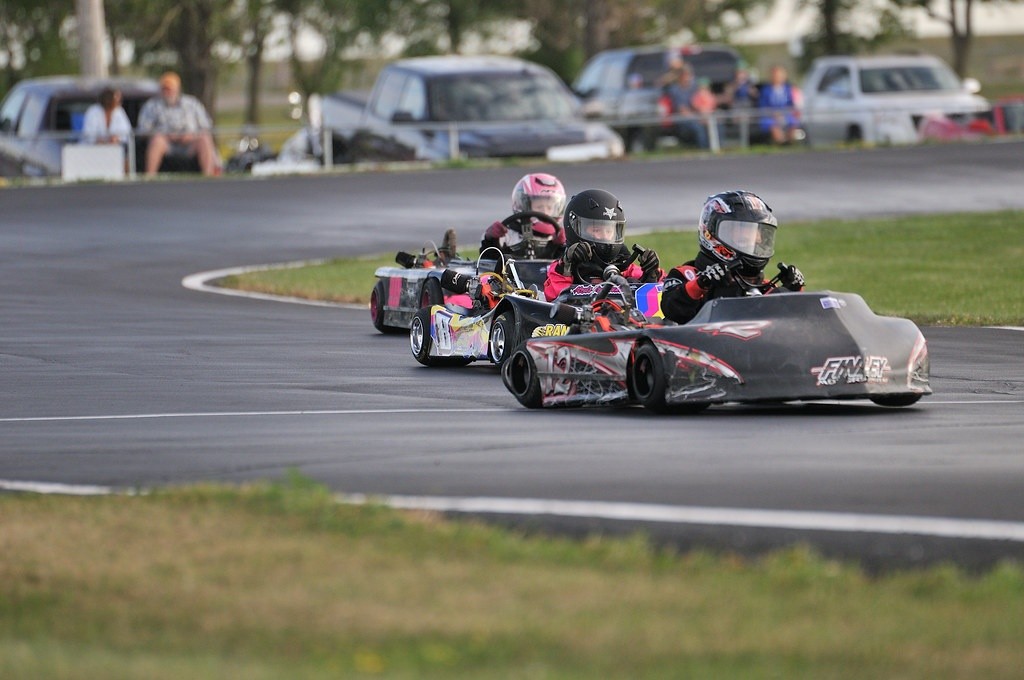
563;189;626;262
512;173;566;234
699;190;778;272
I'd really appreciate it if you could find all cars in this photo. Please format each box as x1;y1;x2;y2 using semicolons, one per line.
792;55;995;145
0;77;223;180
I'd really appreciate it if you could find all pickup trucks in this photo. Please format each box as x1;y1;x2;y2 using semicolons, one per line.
320;55;625;162
571;40;808;151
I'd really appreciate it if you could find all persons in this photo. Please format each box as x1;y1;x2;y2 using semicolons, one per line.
660;189;806;326
757;64;804;144
136;71;215;175
83;86;131;174
480;174;567;278
658;62;759;148
543;189;669;307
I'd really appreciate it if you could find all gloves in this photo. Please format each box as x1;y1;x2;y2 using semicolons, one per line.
638;248;660;275
552;227;566;245
563;241;592;264
486;221;508;241
696;261;732;290
777;262;806;292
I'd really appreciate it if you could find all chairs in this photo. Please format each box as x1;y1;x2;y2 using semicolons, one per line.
633;280;680;326
495;254;561;302
